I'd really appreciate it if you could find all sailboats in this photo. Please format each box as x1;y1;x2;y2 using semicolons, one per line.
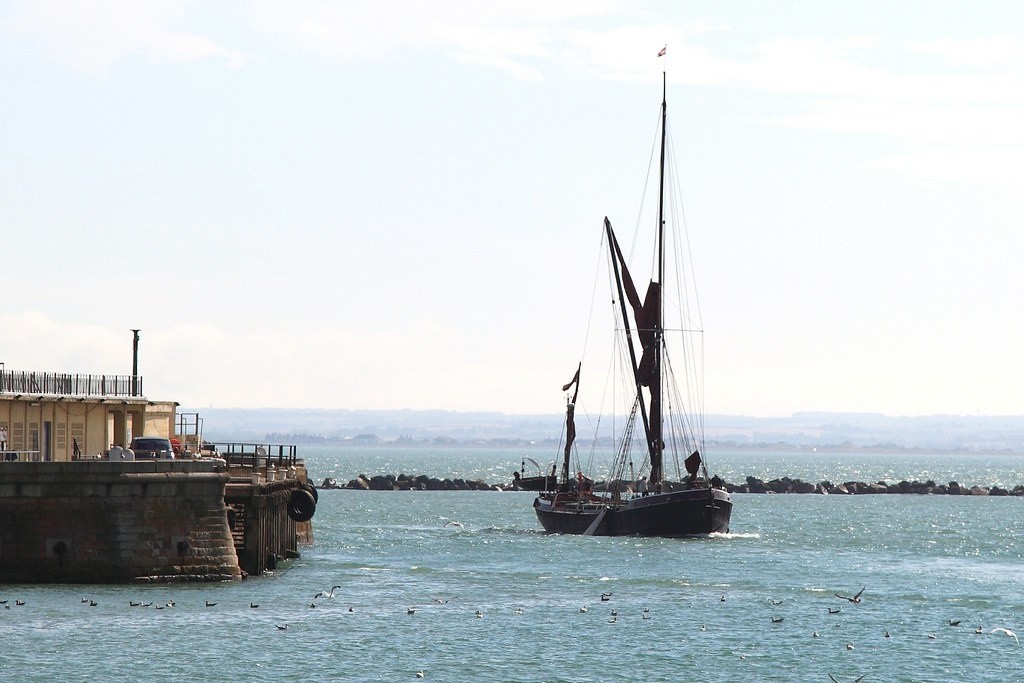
532;43;733;539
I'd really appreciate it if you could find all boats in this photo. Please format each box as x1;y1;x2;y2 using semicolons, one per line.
512;457;558;491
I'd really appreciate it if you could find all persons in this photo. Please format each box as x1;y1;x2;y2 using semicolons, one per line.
639;476;649;497
172;446;220;460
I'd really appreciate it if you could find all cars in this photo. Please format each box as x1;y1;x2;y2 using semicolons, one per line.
342;433;735;448
129;436;177;460
